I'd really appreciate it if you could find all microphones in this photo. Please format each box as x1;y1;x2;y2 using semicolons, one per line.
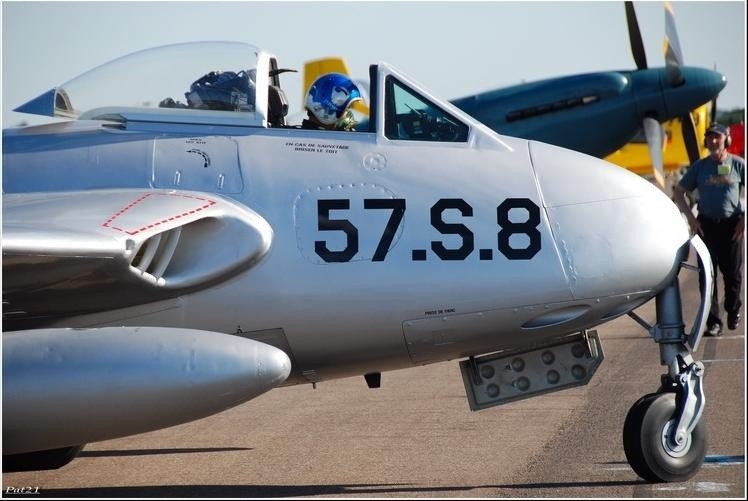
717;147;726;164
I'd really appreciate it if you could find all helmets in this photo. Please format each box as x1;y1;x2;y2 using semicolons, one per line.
303;73;364;126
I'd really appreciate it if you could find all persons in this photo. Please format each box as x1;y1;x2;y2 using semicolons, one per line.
300;72;366;132
674;122;745;337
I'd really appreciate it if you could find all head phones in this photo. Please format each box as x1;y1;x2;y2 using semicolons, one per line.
704;124;732;148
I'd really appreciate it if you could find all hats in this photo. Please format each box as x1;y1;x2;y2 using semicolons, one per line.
704;123;726;135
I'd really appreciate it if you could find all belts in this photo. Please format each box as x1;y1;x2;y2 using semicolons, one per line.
699;212;739;223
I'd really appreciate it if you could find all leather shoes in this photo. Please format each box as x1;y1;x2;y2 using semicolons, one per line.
727;313;741;329
704;324;723;336
